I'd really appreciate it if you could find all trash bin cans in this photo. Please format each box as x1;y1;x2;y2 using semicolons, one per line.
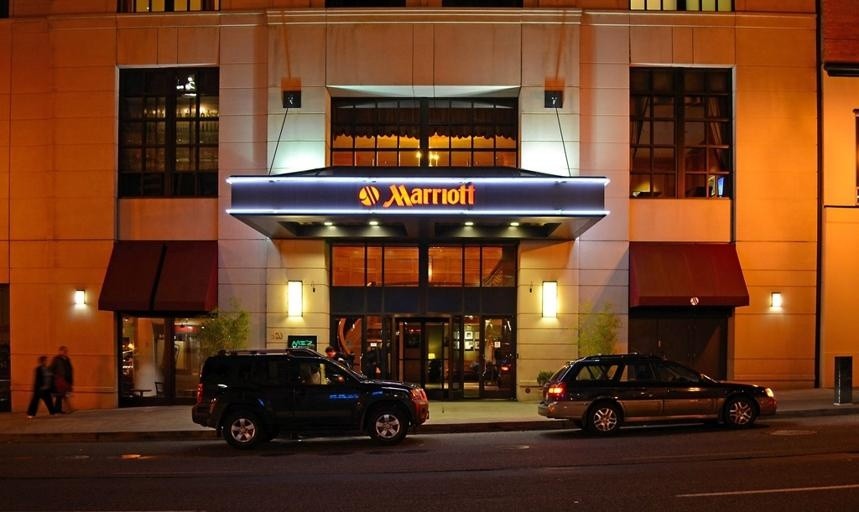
833;356;852;406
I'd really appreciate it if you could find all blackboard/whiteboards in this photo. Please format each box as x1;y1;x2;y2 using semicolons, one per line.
288;336;317;353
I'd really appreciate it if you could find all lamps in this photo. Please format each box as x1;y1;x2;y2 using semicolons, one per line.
542;279;558;318
287;279;303;319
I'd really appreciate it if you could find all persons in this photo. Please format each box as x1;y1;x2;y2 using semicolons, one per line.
324;346;346;383
25;355;56;419
48;346;74;413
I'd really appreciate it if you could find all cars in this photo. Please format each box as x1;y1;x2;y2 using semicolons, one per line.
537;354;776;436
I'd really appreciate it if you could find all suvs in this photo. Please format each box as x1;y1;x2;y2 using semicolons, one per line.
192;349;429;444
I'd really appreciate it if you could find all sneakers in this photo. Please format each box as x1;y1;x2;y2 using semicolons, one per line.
27;411;66;419
